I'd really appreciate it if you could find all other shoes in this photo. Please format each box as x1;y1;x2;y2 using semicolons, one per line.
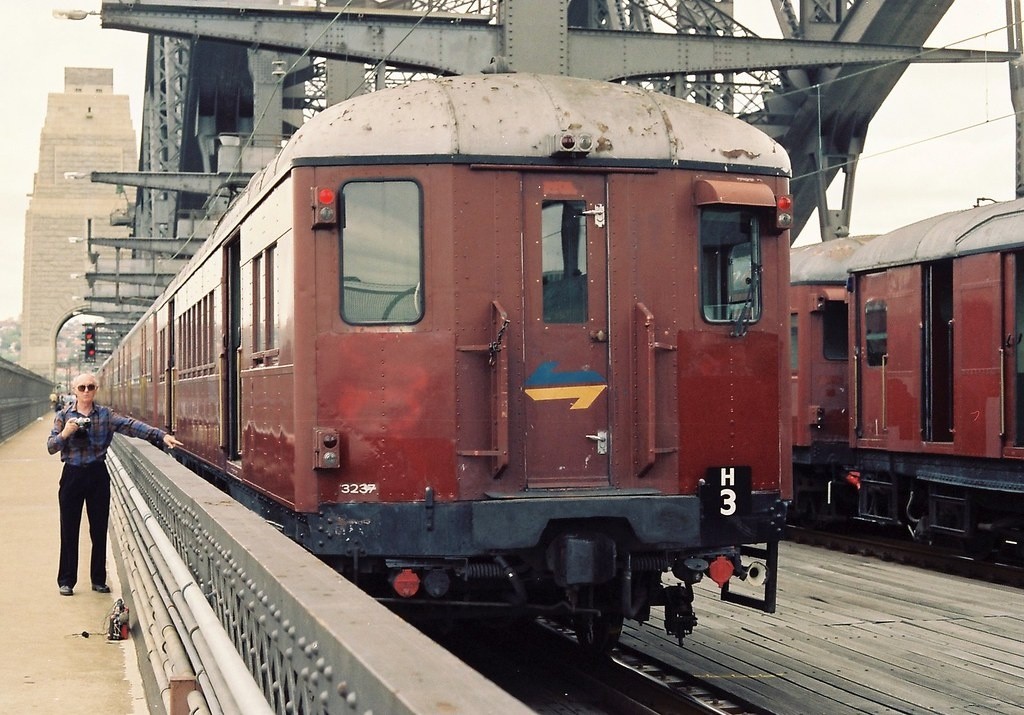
92;584;110;593
60;585;73;595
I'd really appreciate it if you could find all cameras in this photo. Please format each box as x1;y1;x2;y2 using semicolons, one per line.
74;417;91;429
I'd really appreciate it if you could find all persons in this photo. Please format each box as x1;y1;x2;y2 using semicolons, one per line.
49;381;78;413
47;373;184;596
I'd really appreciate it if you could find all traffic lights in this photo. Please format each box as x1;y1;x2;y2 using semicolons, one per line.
85;329;95;359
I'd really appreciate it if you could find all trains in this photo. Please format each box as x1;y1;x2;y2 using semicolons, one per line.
89;72;795;658
791;196;1024;565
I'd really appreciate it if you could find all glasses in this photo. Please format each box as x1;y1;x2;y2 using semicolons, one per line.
77;384;95;392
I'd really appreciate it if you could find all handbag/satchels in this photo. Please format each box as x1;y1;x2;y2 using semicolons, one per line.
108;598;129;640
